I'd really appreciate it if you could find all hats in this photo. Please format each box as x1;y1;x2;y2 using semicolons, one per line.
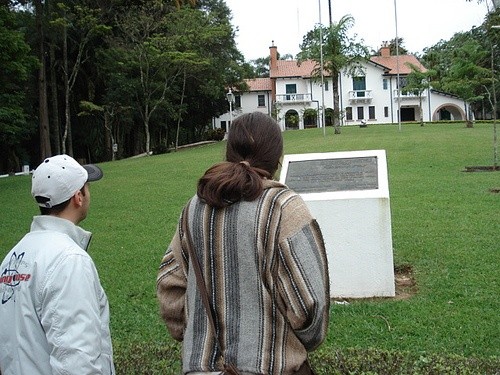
31;154;103;208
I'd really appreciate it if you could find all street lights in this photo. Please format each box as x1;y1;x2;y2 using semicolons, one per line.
227;92;233;126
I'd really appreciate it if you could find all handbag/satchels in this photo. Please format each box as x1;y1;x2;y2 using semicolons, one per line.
219;360;315;375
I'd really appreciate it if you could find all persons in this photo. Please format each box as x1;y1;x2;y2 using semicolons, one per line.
0;154;117;375
155;111;330;375
